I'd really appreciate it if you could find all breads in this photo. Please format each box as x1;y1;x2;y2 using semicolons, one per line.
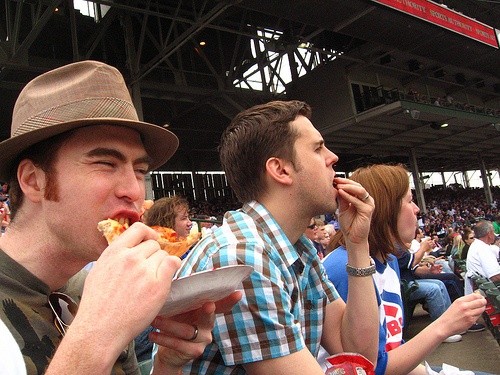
96;218;202;258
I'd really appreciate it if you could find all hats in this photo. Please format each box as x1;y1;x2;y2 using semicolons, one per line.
0;60;179;184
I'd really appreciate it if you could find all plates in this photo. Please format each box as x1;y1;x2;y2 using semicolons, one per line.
157;265;255;318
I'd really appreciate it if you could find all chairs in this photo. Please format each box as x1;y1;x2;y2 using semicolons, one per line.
475;288;500;347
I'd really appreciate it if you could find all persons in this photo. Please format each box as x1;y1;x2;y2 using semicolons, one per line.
319;163;488;375
446;95;454;109
0;182;500;344
408;88;416;103
434;97;441;107
151;100;379;375
0;60;244;374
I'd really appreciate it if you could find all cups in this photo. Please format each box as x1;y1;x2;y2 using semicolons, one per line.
421;237;432;252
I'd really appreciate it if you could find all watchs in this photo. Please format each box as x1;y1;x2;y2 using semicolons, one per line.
345;256;376;277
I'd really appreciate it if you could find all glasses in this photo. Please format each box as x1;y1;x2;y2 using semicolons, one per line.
470;237;474;239
308;223;319;229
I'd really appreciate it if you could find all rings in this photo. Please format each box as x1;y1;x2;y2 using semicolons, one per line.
186;324;198;342
361;192;370;202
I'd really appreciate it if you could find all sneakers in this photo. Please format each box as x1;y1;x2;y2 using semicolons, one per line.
443;334;462;343
468;322;486;333
